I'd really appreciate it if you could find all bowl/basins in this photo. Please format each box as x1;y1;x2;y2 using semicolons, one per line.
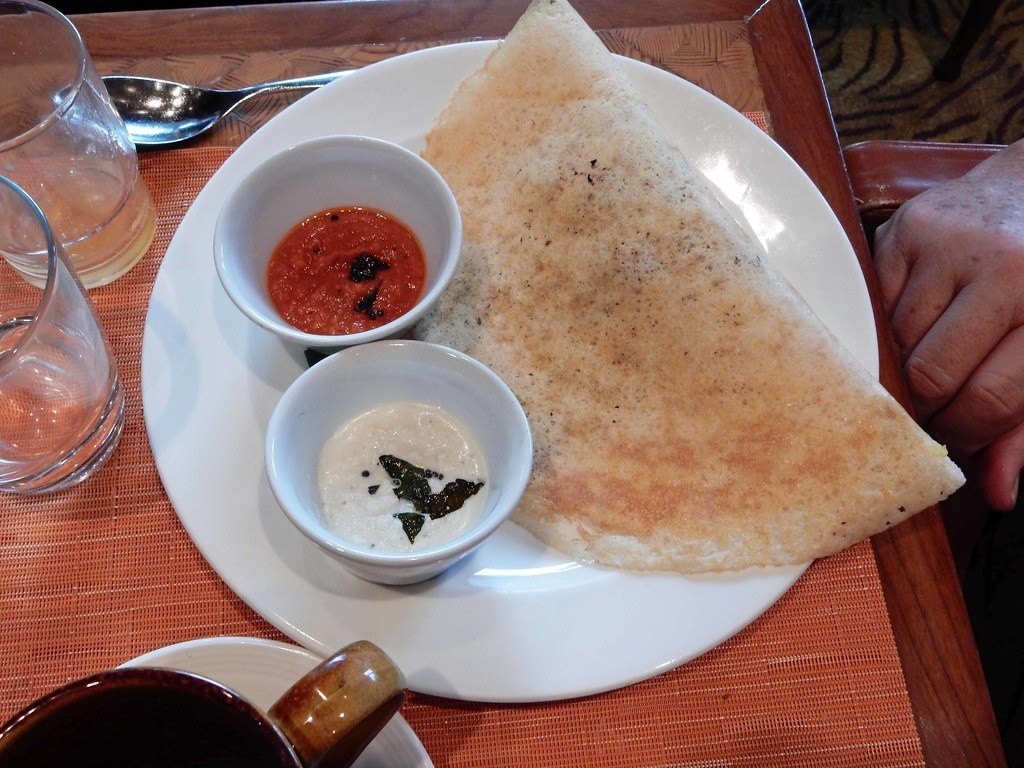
263;339;534;585
214;134;464;356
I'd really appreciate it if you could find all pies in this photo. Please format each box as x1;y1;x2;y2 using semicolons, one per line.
414;0;967;574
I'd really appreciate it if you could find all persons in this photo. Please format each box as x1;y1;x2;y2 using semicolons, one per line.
862;136;1024;514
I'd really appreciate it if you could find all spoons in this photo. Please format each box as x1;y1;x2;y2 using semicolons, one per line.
52;69;359;145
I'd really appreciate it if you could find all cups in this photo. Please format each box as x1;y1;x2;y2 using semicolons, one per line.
0;640;407;768
0;0;158;290
0;176;126;496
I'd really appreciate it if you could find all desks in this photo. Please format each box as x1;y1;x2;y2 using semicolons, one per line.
0;0;1003;768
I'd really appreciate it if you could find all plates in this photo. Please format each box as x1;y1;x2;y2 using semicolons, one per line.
141;40;879;704
116;636;434;768
